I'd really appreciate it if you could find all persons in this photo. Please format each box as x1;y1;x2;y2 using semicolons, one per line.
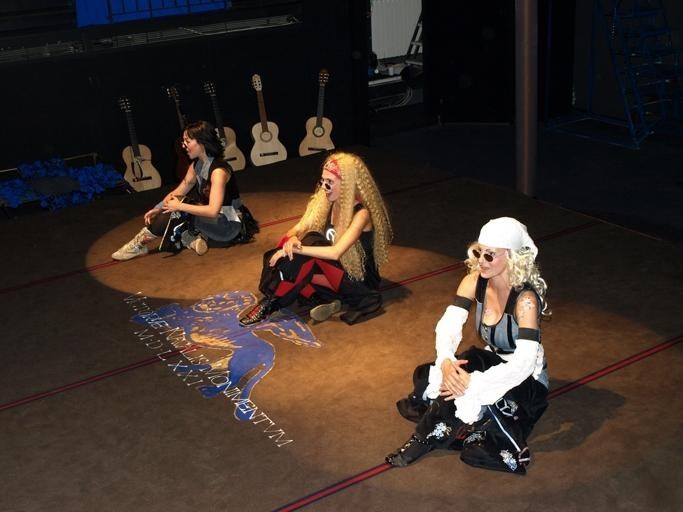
384;216;553;476
238;153;394;328
110;119;258;262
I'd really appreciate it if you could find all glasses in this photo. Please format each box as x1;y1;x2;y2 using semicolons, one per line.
181;142;188;148
318;181;331;189
472;250;505;262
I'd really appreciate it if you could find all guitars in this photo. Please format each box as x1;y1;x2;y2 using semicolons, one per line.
204;79;245;171
165;86;198;184
250;74;288;166
299;70;336;157
120;96;161;193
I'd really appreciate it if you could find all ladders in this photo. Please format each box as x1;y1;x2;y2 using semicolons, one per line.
403;12;424;66
546;0;683;150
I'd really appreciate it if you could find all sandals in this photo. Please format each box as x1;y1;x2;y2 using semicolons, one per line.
385;434;433;466
460;430;526;474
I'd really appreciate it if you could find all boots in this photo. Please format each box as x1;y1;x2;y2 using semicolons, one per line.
307;294;341;321
112;226;159;260
239;295;281;327
181;230;208;255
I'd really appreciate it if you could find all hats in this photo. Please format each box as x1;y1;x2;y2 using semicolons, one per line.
478;217;536;249
